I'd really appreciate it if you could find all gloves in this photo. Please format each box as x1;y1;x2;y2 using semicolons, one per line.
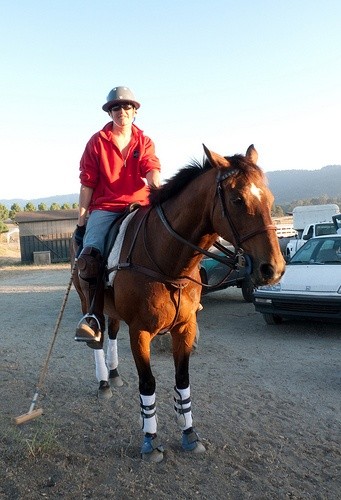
73;224;86;246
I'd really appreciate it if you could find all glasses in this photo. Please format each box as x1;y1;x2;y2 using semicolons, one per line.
111;104;136;112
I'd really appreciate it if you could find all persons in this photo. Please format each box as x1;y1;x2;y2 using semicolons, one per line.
75;87;160;350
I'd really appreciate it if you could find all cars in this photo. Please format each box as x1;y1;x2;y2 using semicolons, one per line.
252;236;341;323
199;237;253;303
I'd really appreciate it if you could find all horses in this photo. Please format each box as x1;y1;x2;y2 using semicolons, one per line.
71;144;286;462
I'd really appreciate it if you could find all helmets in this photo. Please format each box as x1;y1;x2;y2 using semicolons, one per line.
102;86;141;112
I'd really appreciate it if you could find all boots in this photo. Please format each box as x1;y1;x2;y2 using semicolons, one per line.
77;247;104;350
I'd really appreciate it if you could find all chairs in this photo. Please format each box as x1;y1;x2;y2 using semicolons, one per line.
318;249;337;261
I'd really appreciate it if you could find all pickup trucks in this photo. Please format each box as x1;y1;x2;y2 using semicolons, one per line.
286;222;341;262
